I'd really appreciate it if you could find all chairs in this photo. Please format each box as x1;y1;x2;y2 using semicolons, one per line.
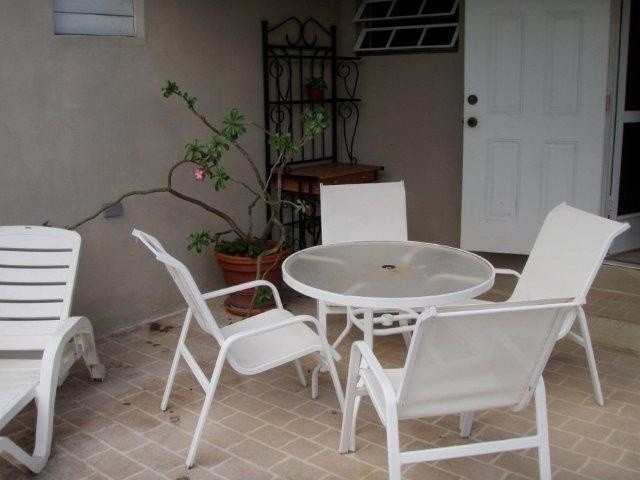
0;226;108;476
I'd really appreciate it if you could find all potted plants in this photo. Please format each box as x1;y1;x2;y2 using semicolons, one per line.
303;74;328;101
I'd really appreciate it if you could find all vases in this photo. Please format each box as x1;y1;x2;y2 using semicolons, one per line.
213;239;288;318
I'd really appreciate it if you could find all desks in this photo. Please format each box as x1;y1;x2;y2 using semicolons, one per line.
273;162;384;252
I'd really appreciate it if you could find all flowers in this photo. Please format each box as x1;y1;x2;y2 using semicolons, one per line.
42;76;332;310
